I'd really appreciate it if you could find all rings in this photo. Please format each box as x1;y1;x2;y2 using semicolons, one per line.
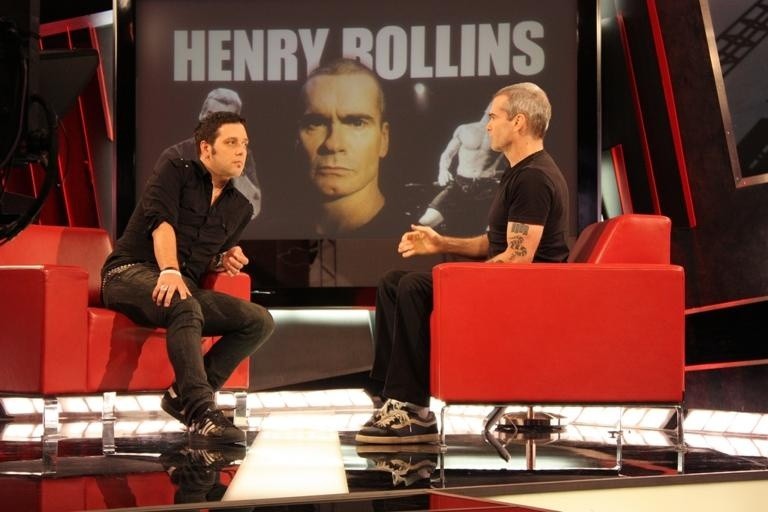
159;282;170;291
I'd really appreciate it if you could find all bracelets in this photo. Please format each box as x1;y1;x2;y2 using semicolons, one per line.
158;267;183;277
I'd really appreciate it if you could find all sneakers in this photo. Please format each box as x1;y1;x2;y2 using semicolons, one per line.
364;399;405;426
161;383;186;426
159;443;246;472
188;406;245;443
355;406;438;444
357;446;440;486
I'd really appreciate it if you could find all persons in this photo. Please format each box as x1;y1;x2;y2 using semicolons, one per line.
293;62;406;238
419;96;523;244
100;112;276;444
357;81;571;446
142;88;265;238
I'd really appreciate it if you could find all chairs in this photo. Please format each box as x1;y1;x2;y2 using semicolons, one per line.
428;213;687;454
0;225;251;433
